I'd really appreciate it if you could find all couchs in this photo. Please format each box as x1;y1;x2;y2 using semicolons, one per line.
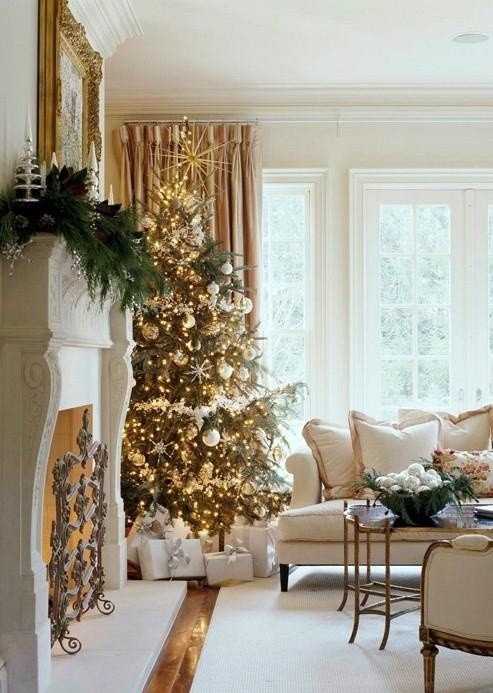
280;407;492;590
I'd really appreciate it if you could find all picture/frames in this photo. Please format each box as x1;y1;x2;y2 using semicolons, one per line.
37;0;103;195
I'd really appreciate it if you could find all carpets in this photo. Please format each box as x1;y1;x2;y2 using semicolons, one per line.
188;571;491;692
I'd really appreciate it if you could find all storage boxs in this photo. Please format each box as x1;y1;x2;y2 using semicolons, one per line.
126;502;279;585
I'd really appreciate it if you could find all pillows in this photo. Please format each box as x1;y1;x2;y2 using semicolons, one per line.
348;409;441;493
397;407;493;452
303;418;359;498
434;447;493;498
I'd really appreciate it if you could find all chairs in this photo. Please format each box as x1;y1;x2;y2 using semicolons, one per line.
419;534;491;692
